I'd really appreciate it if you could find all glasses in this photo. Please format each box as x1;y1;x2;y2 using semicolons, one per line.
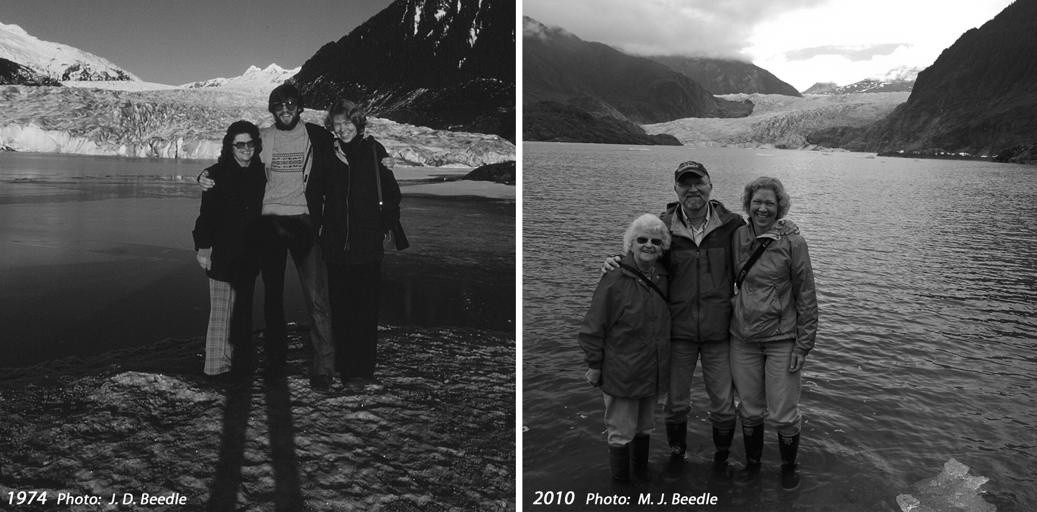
633;236;664;246
231;140;255;150
270;98;298;113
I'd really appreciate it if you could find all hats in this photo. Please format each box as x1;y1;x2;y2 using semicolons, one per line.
673;161;708;182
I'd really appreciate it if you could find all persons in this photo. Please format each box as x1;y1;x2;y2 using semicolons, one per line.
197;79;396;389
577;213;673;492
320;98;402;391
196;120;267;384
600;161;800;478
732;176;819;493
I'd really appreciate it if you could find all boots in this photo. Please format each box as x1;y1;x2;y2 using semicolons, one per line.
630;433;650;483
657;418;687;484
606;443;631;487
777;430;801;492
735;417;762;486
709;421;735;481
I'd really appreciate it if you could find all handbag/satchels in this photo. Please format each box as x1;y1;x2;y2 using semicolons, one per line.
387;206;411;252
